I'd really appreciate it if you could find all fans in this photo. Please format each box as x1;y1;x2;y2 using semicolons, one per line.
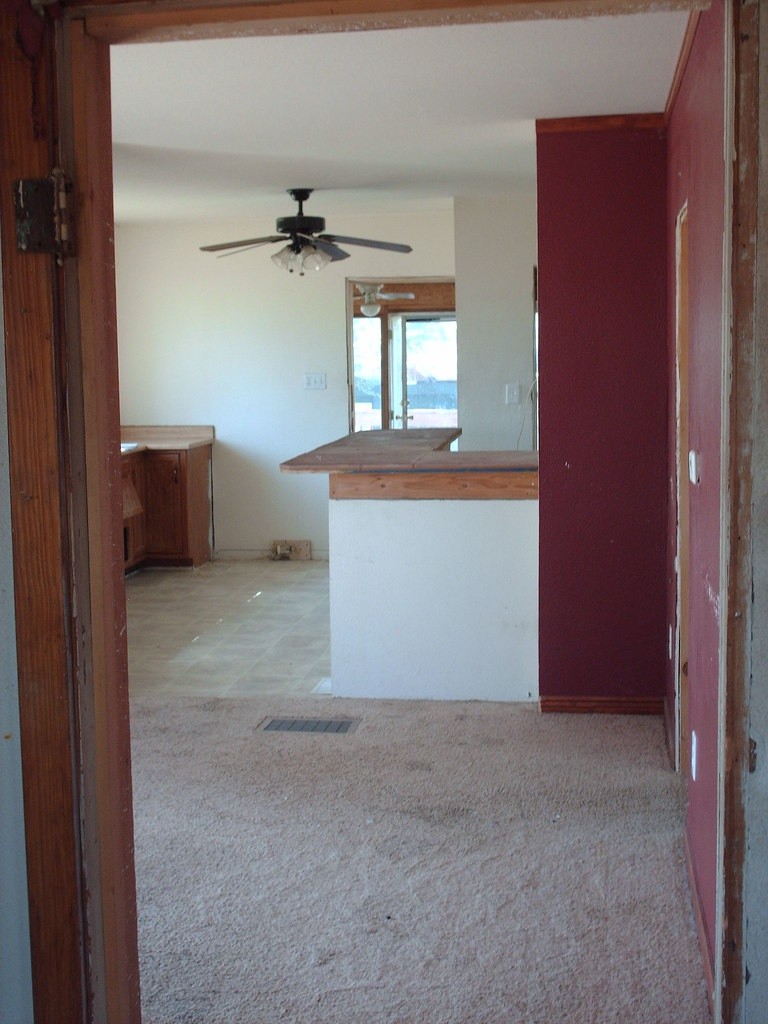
199;189;413;262
353;284;416;300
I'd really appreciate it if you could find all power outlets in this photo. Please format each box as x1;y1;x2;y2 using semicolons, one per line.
691;730;698;782
688;449;700;485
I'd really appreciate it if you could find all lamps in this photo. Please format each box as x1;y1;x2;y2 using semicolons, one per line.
314;247;333;271
271;245;293;270
300;245;322;270
360;295;381;318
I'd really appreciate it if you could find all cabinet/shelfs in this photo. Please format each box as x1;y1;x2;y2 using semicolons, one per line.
145;424;215;567
120;424;145;575
